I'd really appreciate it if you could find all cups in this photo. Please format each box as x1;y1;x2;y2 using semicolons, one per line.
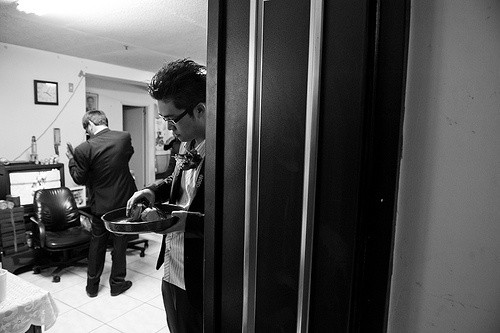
0;269;6;301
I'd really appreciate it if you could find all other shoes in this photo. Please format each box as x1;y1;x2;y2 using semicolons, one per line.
86;286;98;296
110;280;132;296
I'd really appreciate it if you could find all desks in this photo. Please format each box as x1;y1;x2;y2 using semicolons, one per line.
0;268;58;333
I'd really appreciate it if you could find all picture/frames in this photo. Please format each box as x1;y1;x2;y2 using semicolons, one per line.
86;92;99;112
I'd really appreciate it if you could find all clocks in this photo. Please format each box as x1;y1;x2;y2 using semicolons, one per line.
34;80;58;105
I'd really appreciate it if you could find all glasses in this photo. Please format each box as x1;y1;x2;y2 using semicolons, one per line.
85;122;90;136
159;101;199;125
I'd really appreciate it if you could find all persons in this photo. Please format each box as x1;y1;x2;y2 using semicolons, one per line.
65;109;138;297
86;96;95;111
128;57;209;333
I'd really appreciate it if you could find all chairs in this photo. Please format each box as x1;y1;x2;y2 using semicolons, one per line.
29;187;93;282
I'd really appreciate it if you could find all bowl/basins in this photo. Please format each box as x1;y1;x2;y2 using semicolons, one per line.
101;203;186;235
